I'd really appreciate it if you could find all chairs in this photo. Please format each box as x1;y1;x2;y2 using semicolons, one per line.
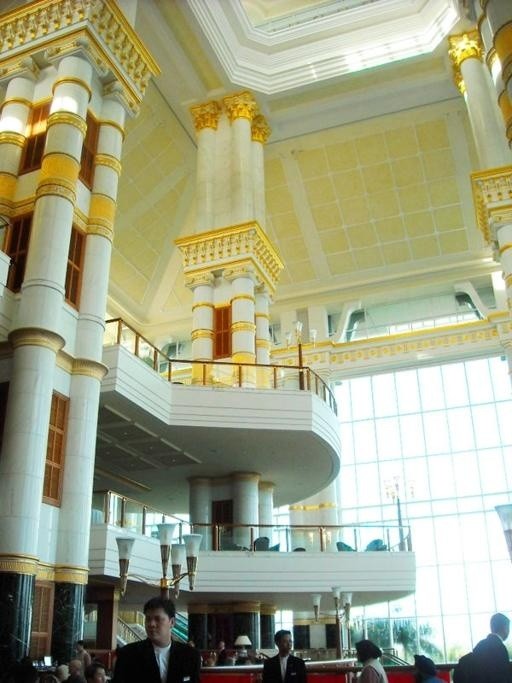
336;538;387;551
221;537;281;551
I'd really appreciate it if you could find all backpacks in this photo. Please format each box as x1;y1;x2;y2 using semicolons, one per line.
453;653;484;683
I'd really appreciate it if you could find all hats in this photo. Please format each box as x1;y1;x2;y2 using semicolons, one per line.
414;655;435;674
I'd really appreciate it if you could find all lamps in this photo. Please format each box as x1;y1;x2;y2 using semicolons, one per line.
234;635;252;657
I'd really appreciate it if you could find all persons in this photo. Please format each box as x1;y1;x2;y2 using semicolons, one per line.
355;640;389;683
113;597;201;683
262;630;307;683
413;654;448;683
216;640;227;666
14;640;109;683
473;613;512;683
